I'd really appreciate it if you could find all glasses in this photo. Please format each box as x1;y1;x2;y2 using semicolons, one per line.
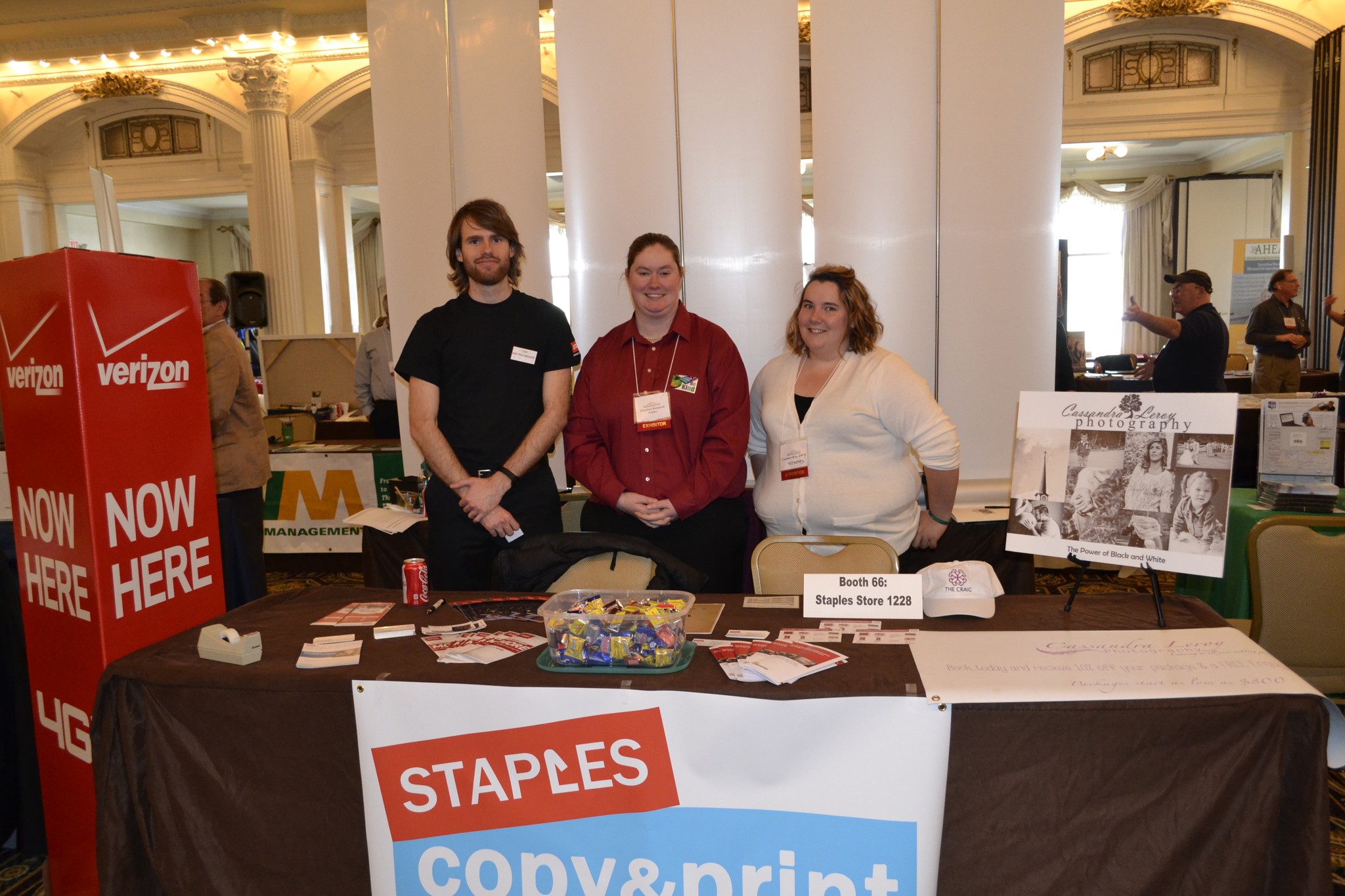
1279;280;1300;284
1168;287;1200;296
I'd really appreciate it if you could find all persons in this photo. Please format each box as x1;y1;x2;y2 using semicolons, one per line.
1122;270;1229;393
1023;505;1060;539
563;230;749;595
1079;469;1123;545
1121;518;1146;548
1173;471;1224;556
1012;499;1037;536
750;264;960;575
1244;270;1311;395
1124;437;1172;513
1055;276;1076;392
1184;436;1228;465
396;199;578;594
198;278;272;613
1070;468;1116;537
354;294;400;439
1076;433;1090;468
1323;294;1345;430
1142;520;1163;550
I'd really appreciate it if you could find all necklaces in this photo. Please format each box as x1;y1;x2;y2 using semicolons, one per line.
639;332;663;341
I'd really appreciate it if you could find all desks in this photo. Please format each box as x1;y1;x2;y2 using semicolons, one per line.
262;439;405;554
1233;391;1345;488
89;588;1332;895
1073;370;1340;395
1176;488;1345;618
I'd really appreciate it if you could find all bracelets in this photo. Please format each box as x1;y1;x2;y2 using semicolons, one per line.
929;509;951;525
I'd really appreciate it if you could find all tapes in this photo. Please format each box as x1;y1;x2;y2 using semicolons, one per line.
218;627;240;647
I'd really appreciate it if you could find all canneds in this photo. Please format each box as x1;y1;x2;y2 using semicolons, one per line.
400;557;429;606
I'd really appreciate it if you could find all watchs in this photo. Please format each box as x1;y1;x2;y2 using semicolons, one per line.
497;466;519;487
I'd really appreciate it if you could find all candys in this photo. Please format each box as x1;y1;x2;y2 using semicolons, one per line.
548;595;687;667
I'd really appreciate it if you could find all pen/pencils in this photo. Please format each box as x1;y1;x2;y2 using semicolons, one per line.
426;598;446;614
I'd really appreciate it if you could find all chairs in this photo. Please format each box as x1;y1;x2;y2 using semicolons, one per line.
750;535;901;594
1247;516;1345;704
545;551;658;591
1225;353;1249;371
1092;354;1140;374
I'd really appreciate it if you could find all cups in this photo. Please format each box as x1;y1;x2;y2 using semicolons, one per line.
310;390;322;410
340;402;349;416
281;426;293;444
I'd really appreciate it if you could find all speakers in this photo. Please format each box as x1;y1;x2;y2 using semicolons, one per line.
224;269;269;328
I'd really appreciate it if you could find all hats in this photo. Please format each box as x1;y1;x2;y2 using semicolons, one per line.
1163;269;1212;294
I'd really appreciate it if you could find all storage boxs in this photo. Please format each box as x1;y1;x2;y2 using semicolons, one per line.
537;588;698;668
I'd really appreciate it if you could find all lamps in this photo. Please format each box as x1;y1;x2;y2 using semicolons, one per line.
1086;144;1129;162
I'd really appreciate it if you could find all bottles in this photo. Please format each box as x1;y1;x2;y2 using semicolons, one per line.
311;404;318;418
